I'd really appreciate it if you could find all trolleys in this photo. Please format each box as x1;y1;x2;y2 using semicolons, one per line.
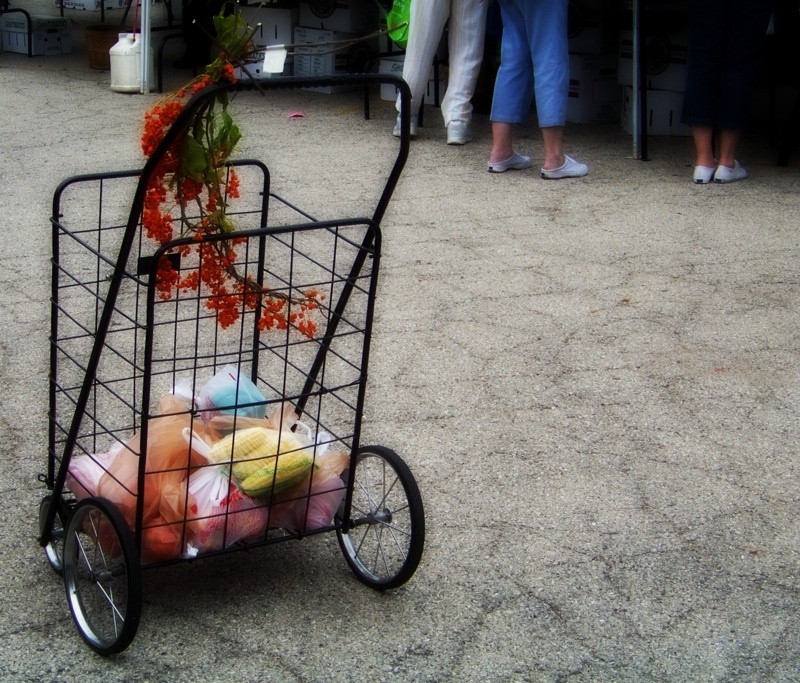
35;71;426;657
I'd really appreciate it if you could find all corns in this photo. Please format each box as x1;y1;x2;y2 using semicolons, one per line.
213;426;312;496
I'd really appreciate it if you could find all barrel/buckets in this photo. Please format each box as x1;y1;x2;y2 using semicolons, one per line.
84;24;138;70
109;32;156;92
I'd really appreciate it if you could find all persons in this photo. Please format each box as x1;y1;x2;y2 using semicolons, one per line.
679;0;772;184
487;0;589;180
393;0;488;145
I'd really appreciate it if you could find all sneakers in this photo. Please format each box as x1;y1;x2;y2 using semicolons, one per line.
446;121;472;145
714;160;748;181
392;114;417;136
692;165;716;183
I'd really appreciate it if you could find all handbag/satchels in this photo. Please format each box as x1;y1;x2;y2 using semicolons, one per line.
67;369;351;557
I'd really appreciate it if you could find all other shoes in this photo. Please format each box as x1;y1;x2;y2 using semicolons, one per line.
541;152;588;179
487;151;532;171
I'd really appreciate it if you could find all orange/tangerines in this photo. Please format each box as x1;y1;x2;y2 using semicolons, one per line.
94;467;198;559
